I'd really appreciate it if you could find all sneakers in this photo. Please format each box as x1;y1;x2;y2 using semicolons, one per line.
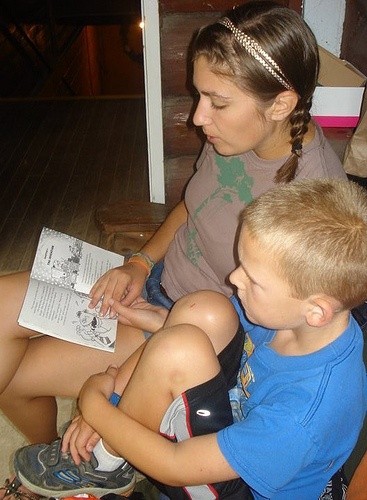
11;437;137;499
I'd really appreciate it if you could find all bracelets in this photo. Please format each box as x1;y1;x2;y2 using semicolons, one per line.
128;252;154;278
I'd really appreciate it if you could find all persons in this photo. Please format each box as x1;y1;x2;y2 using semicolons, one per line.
12;178;367;500
0;1;350;500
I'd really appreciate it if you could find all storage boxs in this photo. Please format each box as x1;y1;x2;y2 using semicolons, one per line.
309;45;367;127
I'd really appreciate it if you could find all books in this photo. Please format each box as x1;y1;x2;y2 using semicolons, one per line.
17;227;125;353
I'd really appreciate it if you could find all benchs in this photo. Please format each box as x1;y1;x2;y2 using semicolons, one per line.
93;174;366;500
